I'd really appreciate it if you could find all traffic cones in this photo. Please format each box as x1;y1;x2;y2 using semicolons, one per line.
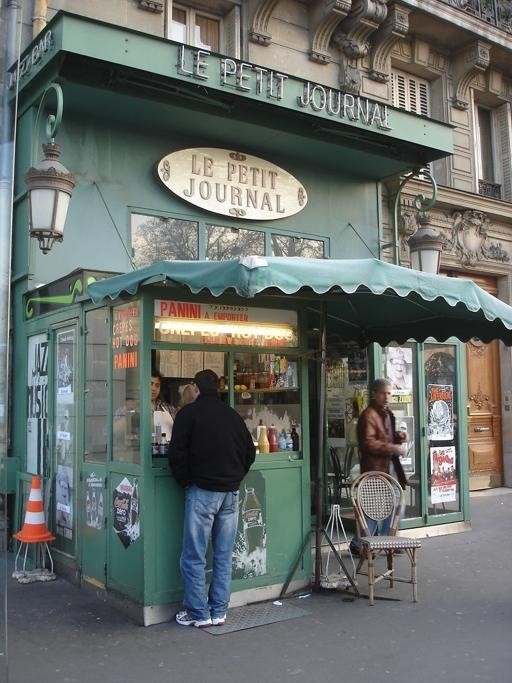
9;473;59;545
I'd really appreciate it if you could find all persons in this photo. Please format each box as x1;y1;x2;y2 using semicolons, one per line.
387;352;411;389
350;378;410;560
168;368;255;628
431;464;456;484
102;369;181;456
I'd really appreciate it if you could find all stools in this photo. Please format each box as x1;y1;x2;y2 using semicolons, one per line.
12;534;58;585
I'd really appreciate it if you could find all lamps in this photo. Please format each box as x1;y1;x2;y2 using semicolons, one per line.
391;169;443;273
26;88;79;252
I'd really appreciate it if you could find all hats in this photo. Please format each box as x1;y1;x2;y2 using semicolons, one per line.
388;347;405;361
192;369;219;390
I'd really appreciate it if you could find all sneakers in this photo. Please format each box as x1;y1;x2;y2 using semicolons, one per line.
387;548;406;556
175;611;227;628
347;544;377;560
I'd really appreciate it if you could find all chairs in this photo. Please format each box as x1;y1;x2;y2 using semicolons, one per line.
350;470;421;606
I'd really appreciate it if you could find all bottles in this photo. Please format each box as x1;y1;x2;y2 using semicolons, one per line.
130;478;139;526
152;432;168;458
242;481;264;554
254;426;299;454
237;355;295;389
